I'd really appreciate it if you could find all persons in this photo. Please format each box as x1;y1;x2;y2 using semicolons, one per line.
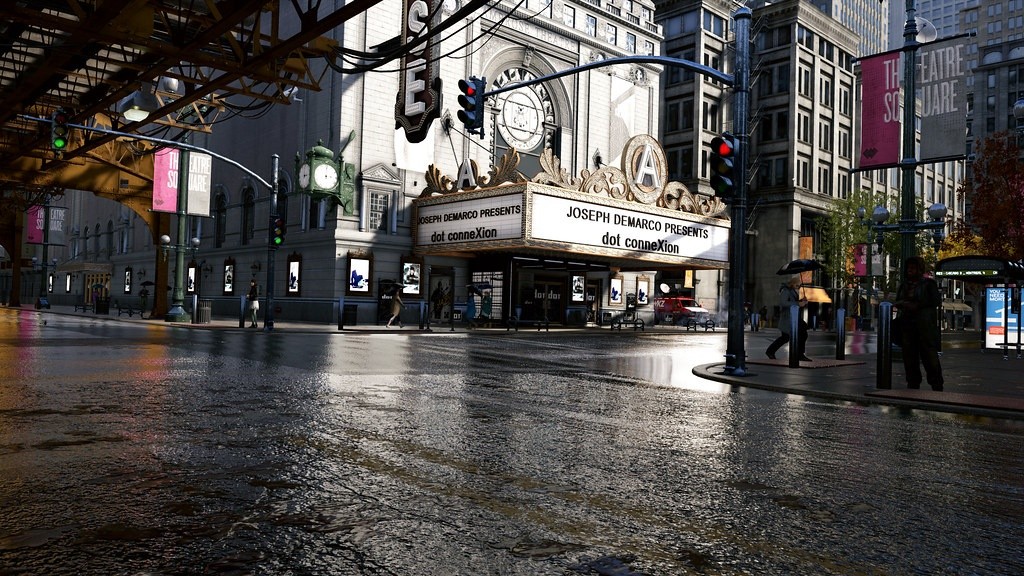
247;279;259;328
385;288;406;328
894;256;945;392
481;292;492;323
430;281;445;319
465;291;478;330
766;277;812;362
760;306;767;329
140;286;148;303
93;288;99;305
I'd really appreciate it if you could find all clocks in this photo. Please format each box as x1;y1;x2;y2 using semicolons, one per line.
297;163;311;188
314;164;338;189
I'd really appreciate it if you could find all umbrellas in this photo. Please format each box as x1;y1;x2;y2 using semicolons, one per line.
92;284;104;288
776;259;823;297
140;281;154;286
464;285;483;297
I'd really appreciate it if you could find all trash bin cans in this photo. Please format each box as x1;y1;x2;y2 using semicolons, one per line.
344;304;359;325
862;317;871;331
95;295;111;315
197;298;213;324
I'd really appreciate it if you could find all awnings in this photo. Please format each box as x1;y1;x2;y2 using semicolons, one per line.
942;302;972;311
799;287;831;303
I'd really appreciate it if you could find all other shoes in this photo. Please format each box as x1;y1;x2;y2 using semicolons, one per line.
400;324;406;328
472;322;478;328
800;355;812;362
466;326;472;330
765;350;776;359
488;322;493;328
385;324;391;328
483;323;487;328
249;323;258;328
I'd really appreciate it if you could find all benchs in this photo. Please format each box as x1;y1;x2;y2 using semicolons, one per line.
119;305;145;318
507;316;715;332
75;303;94;313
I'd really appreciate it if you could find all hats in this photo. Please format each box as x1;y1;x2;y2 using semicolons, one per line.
787;276;803;285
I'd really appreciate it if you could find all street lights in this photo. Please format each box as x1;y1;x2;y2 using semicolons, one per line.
870;1;949;298
30;192;58;310
157;68;213;325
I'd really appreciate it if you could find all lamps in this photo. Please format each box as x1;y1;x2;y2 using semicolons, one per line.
137;267;146;280
203;263;213;279
72;273;79;283
251;259;262;276
120;88;158;122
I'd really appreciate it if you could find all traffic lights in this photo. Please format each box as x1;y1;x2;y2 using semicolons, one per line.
457;78;486;129
52;110;69;150
709;133;739;198
269;215;285;247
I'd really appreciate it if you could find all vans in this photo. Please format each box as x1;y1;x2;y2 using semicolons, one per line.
653;297;710;326
